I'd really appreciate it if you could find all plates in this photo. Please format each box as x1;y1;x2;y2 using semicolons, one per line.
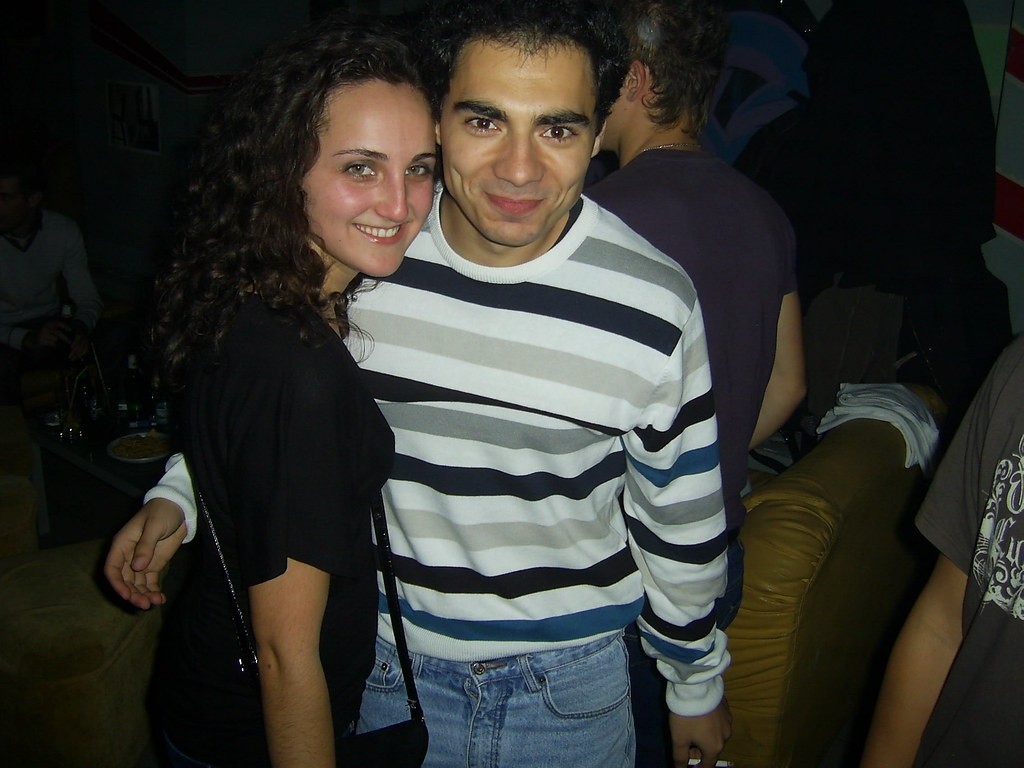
107;432;171;464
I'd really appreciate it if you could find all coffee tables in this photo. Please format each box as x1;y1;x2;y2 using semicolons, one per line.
26;408;177;543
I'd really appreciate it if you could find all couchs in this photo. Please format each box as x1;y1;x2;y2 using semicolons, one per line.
21;301;135;414
722;377;948;768
1;403;171;768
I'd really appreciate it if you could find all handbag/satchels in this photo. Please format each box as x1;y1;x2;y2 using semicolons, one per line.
336;717;428;768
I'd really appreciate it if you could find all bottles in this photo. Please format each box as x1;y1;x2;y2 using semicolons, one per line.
126;352;148;426
155;392;177;433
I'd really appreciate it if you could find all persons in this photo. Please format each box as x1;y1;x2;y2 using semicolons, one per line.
105;0;734;767
577;1;810;767
0;157;106;531
149;26;438;767
868;335;1024;768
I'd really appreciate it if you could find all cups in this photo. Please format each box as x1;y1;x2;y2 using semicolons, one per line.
60;392;83;440
